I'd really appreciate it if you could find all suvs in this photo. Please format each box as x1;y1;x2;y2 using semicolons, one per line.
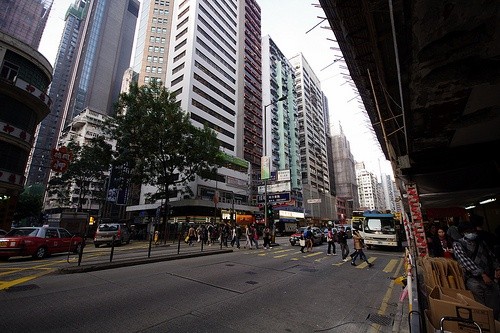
94;223;131;247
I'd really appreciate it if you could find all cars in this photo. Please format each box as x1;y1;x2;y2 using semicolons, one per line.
289;226;325;247
324;224;352;242
0;224;86;261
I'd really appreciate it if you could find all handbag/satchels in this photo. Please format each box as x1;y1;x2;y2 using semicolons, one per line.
184;236;189;243
327;236;333;242
299;240;305;246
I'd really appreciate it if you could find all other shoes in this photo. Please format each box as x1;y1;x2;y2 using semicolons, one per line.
300;250;305;253
270;247;272;249
307;251;309;252
264;247;267;249
360;257;363;260
351;262;357;266
368;263;375;267
327;253;331;256
342;258;349;262
311;251;314;253
333;253;337;256
244;246;246;249
350;254;353;259
249;248;252;249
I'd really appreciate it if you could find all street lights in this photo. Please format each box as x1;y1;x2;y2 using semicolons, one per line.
264;94;289;248
214;164;223;241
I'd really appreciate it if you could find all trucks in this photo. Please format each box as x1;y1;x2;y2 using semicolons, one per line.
361;213;399;249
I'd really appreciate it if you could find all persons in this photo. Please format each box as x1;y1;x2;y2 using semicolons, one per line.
244;224;260;249
338;227;349;261
218;224;230;248
327;227;337;255
350;230;373;267
196;224;216;246
230;224;242;249
188;225;196;246
428;214;500;310
300;226;316;253
263;226;273;249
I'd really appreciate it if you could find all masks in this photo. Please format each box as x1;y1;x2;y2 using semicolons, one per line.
463;232;478;241
